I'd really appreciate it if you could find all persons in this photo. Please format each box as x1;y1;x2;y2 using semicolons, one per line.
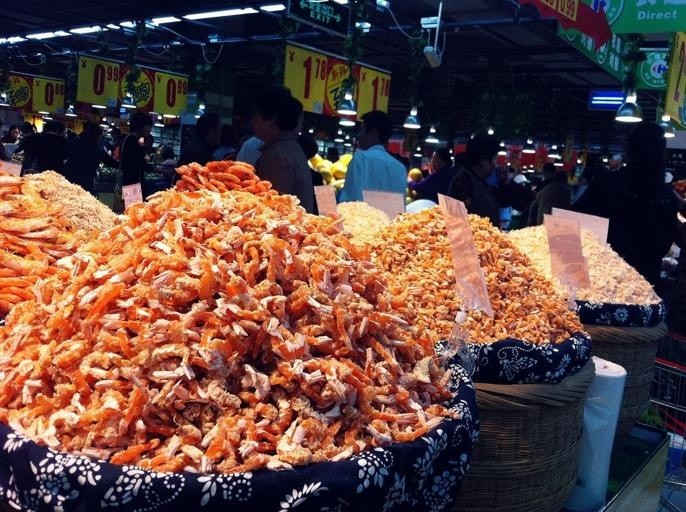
327;147;337;163
604;120;686;293
0;86;152;219
408;148;608;230
176;86;324;215
339;110;406;210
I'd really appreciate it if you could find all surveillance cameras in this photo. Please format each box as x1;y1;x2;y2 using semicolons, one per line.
424;46;442;68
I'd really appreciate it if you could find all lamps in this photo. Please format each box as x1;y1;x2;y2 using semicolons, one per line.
0;87;167;128
333;88;565;169
615;88;677;140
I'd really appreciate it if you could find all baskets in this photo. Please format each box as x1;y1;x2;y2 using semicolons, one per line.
445;354;595;511
583;321;670;433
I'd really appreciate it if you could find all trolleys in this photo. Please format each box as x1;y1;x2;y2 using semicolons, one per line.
645;331;685;512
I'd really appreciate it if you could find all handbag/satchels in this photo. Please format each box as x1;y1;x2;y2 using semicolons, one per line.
112;186;125;214
